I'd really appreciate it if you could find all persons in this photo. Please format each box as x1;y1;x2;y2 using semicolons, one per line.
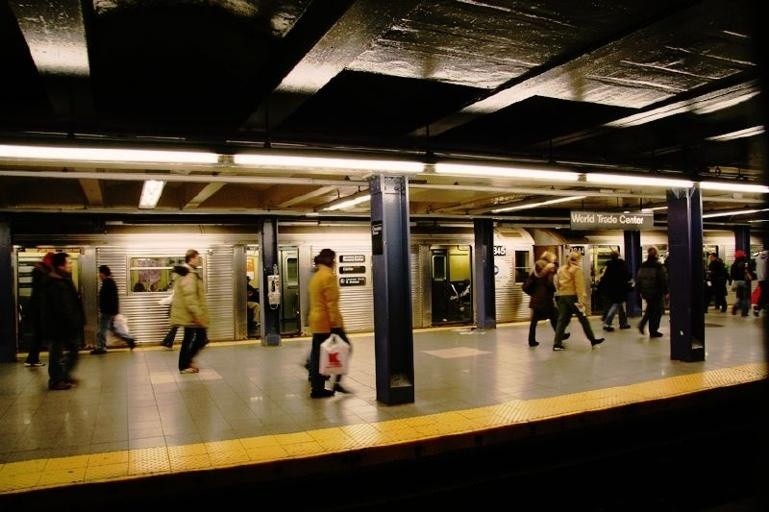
704;245;769;317
305;248;353;398
521;248;670;351
23;252;260;390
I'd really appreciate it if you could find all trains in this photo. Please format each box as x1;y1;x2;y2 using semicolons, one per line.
15;227;765;360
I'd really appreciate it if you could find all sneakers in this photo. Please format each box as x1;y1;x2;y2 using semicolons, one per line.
310;389;333;398
331;382;355;396
528;322;665;352
50;376;79;390
23;360;46;368
129;338;137;350
89;348;108;354
180;366;200;374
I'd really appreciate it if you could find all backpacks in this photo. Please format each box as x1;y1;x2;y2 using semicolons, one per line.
637;264;660;299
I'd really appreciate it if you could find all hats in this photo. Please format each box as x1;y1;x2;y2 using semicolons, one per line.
648;248;657;255
734;248;747;257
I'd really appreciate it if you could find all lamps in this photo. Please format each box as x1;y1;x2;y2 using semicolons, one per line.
702;204;769;218
314;186;370;211
640;198;667;213
137;181;166;210
492;197;585;214
